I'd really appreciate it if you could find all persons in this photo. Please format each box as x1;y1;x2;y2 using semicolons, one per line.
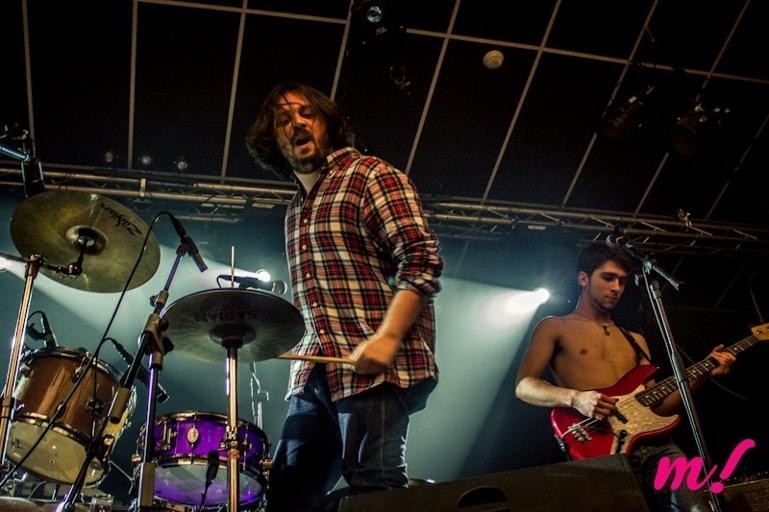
512;241;717;511
242;77;456;510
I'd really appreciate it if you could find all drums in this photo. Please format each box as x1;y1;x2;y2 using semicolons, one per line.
5;343;137;488
132;410;273;510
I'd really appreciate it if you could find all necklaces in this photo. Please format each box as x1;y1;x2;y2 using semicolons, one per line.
572;311;614;336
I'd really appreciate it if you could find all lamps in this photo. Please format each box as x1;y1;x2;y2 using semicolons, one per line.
598;77;659;141
674;96;733;138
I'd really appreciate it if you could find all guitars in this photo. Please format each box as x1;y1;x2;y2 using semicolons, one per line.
552;324;768;462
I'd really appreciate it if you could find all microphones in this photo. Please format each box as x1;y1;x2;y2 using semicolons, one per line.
171;215;209;272
115;343;170;404
605;233;637;259
234;277;289;296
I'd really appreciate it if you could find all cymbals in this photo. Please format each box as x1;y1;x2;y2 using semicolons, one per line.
159;288;306;362
10;189;160;292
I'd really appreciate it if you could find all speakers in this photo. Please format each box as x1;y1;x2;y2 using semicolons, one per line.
337;452;651;511
